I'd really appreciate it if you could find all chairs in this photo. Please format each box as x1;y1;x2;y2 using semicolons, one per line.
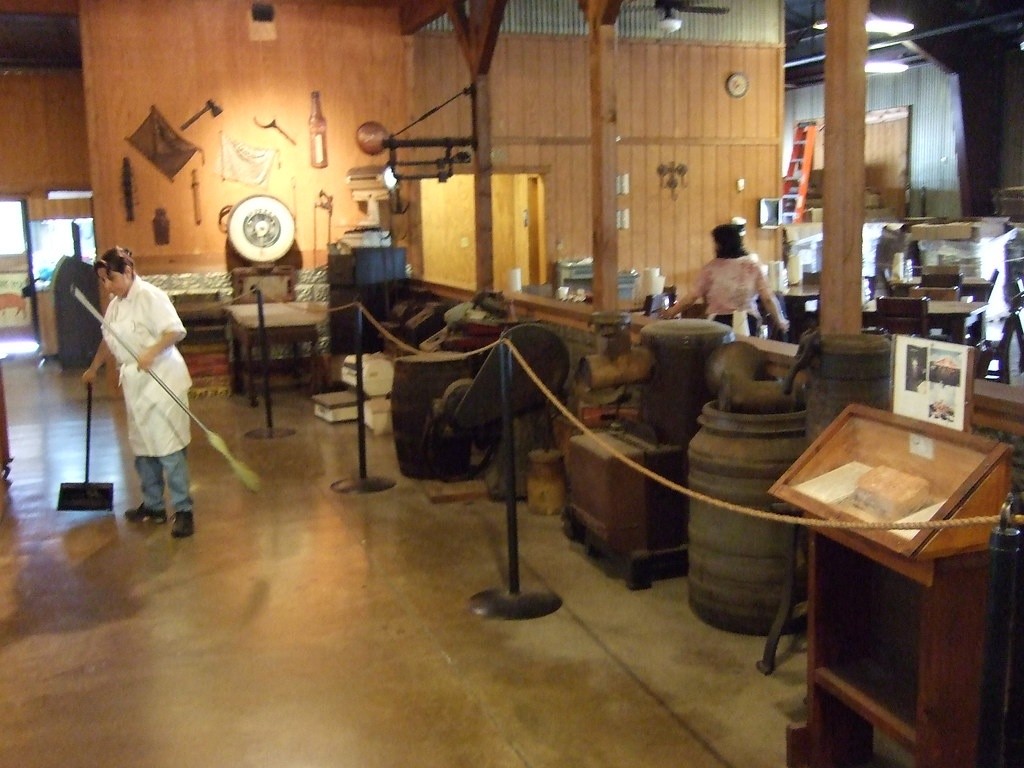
880;268;896;296
875;294;932;338
230;267;320;409
964;291;1024;384
941;268;1000;340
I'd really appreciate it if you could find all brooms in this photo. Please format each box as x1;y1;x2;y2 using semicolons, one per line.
73;286;262;496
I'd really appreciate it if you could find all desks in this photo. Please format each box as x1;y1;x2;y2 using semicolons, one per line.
768;284;820;345
886;276;994;339
862;299;990;346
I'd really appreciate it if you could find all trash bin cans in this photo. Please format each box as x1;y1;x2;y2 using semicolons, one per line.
51;255;104;371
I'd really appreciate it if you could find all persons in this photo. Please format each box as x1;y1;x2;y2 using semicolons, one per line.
82;248;194;537
657;225;790;338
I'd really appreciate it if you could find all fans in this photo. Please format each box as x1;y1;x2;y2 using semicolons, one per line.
622;0;729;14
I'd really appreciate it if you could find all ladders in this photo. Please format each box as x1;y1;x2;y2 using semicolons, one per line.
782;121;820;225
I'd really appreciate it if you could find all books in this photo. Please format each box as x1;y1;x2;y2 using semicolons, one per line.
892;335;969;432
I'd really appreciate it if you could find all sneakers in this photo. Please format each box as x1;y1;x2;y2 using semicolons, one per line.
124;502;167;522
169;510;194;537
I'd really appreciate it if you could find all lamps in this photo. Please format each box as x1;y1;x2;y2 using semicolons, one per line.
658;8;683;34
